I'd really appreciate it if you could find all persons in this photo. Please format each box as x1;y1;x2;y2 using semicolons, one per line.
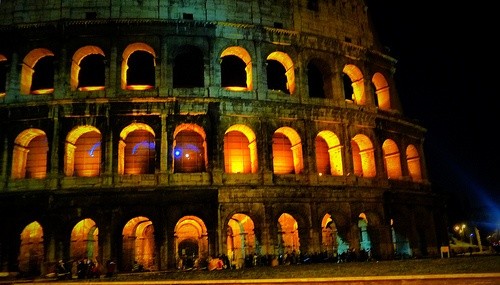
19;247;370;282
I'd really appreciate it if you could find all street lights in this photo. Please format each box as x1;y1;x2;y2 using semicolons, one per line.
218;203;227;258
